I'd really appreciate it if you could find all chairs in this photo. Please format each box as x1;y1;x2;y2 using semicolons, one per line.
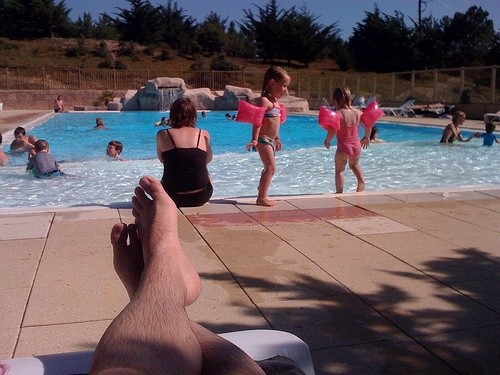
324;99;500;124
0;330;314;375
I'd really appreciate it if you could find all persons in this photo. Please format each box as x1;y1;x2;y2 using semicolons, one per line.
479;122;500;146
155;97;212;207
0;132;9;167
10;127;37;152
26;140;65;179
54;95;64;113
441;111;479;143
319;86;386;193
235;67;291;207
95;118;105;127
90;176;264;375
201;111;207;119
154;117;170;126
106;141;126;161
226;113;237;122
